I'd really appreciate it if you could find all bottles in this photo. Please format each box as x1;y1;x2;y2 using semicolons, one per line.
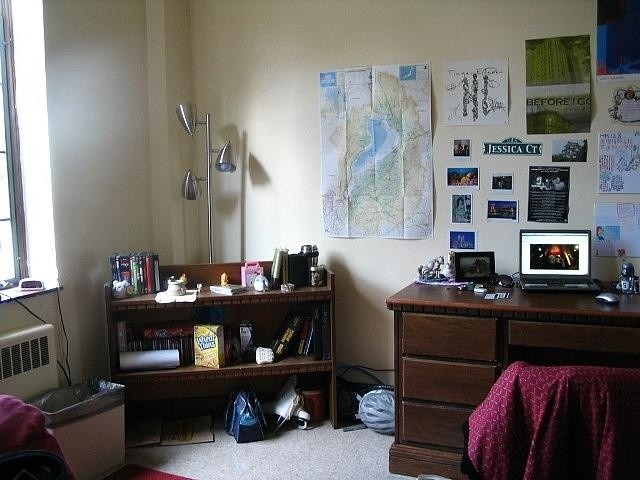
619;262;636;296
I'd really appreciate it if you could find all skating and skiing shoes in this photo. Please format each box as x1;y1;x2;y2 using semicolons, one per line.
290;394;313;431
259;379;303;435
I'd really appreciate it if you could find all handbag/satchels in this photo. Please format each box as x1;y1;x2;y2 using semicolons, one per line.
223;383;271;445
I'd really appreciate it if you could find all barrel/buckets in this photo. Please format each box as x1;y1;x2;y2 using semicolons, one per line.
298;384;328;424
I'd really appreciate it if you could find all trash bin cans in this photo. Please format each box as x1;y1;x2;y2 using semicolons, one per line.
24;379;127;480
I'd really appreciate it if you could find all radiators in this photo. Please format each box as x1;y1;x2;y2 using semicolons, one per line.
0;324;61;404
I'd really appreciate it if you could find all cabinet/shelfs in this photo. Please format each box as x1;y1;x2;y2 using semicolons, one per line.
103;257;341;429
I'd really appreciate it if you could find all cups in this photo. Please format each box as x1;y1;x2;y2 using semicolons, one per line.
166;278;187;296
301;245;325;286
281;283;295;292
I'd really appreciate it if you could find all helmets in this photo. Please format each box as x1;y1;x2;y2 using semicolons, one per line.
358;388;396;436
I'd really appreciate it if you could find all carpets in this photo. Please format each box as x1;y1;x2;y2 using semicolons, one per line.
103;462;196;479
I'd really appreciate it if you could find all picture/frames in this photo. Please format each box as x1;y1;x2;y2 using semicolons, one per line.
453;251;496;281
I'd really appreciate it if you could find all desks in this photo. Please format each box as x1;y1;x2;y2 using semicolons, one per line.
383;274;640;480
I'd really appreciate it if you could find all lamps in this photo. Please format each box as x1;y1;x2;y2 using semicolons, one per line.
172;103;239;265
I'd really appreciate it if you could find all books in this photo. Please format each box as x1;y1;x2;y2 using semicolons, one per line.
267;302;330;361
119;313;208;365
265;246;310;287
109;251;163;293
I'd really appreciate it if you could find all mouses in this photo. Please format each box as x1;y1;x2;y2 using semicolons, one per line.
595;292;620;304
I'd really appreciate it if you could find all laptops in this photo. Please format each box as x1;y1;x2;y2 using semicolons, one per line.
520;229;602;292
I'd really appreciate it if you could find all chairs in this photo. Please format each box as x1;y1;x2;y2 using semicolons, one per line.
462;360;640;480
